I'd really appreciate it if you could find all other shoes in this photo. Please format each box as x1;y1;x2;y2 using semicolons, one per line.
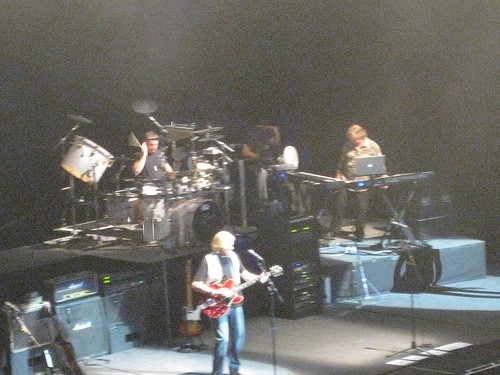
357;231;365;240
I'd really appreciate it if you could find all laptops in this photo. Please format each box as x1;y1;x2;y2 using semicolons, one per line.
349;155;387;177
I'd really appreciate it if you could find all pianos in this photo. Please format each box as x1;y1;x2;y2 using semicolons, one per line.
287;170;347;244
345;154;436;249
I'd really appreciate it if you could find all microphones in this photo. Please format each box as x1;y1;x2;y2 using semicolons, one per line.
390;221;408;231
247;249;264;264
3;301;23;316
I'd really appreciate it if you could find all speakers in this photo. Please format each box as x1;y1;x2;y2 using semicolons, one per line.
0;285;152;375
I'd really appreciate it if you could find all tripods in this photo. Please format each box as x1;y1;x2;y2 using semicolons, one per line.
386;230;443;362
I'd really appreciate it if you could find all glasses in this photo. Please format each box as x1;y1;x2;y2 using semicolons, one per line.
144;138;159;141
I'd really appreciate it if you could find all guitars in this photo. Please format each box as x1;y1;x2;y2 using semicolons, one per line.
179;256;206;337
4;301;57;375
42;306;83;375
198;265;285;318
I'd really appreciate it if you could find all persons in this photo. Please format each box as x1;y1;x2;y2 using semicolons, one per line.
242;125;297;214
132;131;177;254
191;231;269;375
326;125;389;241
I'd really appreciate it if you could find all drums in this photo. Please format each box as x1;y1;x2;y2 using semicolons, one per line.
61;135;113;185
109;166;229;245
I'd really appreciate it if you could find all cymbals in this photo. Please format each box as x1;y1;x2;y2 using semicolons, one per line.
193;126;225;134
197;134;227;142
